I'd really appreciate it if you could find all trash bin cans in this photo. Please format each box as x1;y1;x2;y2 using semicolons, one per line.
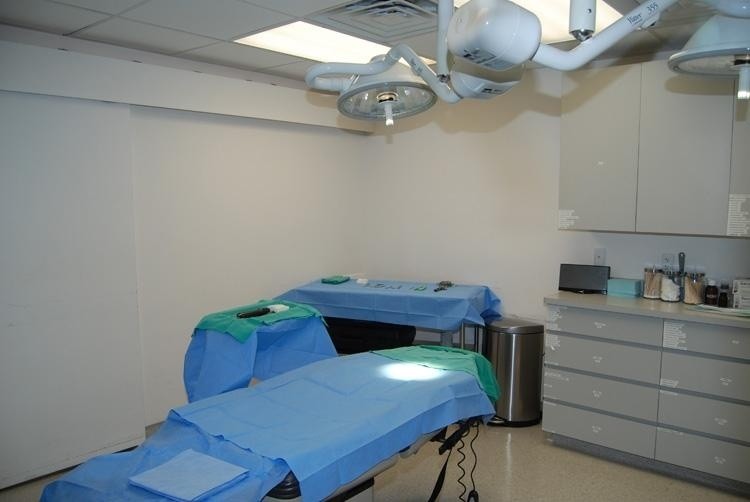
489;317;543;428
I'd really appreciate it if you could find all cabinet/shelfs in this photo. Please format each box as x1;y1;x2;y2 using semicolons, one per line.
556;61;749;239
543;289;749;497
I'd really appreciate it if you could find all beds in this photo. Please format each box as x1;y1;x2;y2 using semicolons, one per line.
39;339;503;500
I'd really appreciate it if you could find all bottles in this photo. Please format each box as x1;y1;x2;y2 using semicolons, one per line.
643;267;729;307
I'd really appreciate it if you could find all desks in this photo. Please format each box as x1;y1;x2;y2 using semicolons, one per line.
274;275;504;349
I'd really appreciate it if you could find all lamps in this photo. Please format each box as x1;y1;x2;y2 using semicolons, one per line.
304;1;750;127
